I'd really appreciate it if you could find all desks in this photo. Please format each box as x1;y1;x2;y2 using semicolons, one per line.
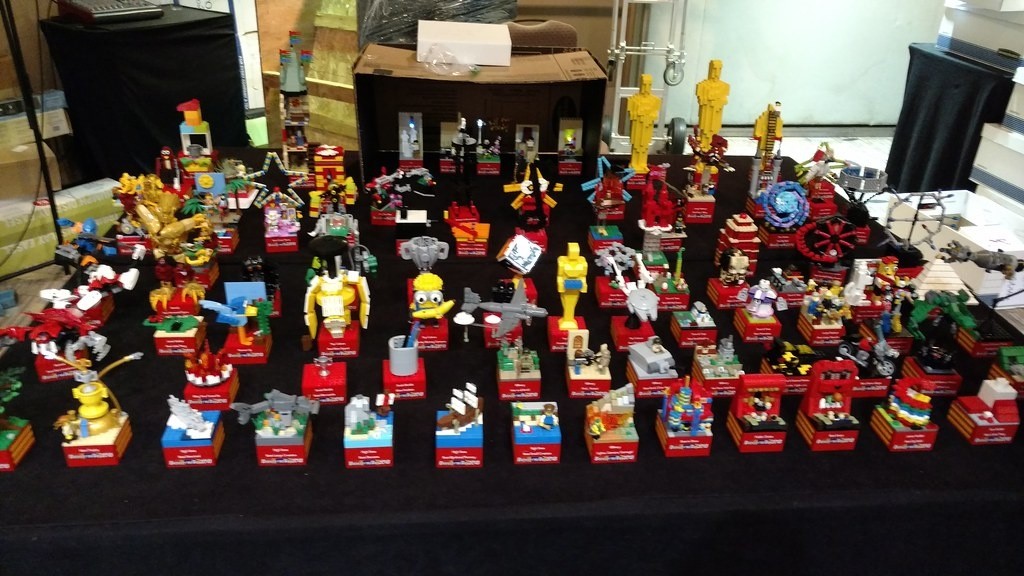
0;154;1024;576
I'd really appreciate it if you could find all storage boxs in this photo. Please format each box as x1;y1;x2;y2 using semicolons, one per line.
351;42;609;187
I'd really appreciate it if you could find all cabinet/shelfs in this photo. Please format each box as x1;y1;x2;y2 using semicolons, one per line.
0;0;71;280
